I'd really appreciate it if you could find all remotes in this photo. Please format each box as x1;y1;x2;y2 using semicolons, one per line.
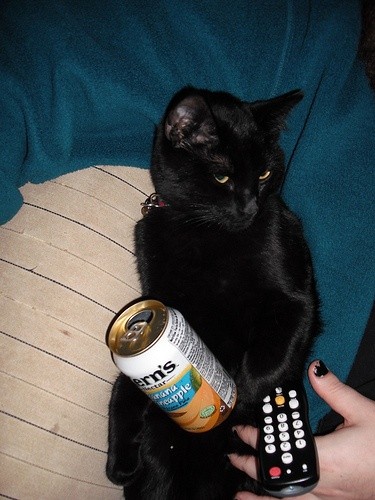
255;378;320;497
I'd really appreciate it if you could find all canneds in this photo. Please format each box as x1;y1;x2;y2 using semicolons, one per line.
107;300;237;435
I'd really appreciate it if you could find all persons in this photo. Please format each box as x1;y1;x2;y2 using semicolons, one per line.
224;357;375;500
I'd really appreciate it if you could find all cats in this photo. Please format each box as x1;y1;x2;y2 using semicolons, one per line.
103;82;327;500
